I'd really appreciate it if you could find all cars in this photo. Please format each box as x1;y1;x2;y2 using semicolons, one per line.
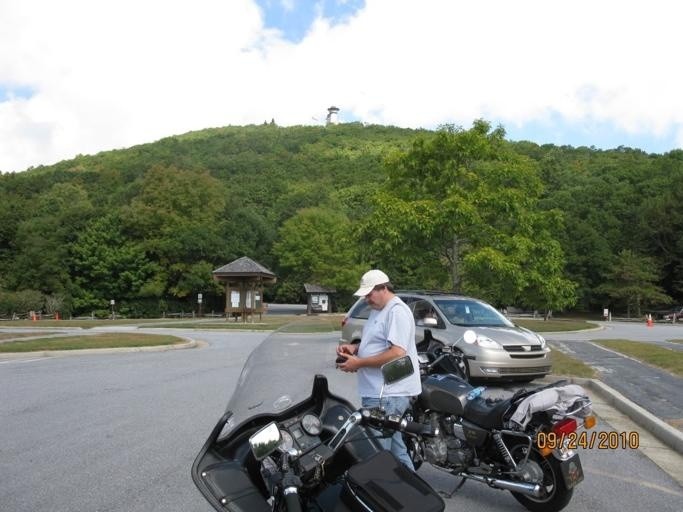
338;290;553;386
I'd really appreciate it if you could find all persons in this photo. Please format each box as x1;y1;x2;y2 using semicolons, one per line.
453;306;468;325
336;269;423;473
417;307;437;326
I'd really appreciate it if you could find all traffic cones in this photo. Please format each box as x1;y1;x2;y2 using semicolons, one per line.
646;313;653;327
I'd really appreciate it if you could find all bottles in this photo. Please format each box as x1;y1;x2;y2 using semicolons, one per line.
465;385;488;401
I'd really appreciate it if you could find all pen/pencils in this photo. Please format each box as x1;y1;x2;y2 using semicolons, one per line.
336;351;348;368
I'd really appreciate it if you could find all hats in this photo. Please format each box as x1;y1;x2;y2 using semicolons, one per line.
351;269;390;297
